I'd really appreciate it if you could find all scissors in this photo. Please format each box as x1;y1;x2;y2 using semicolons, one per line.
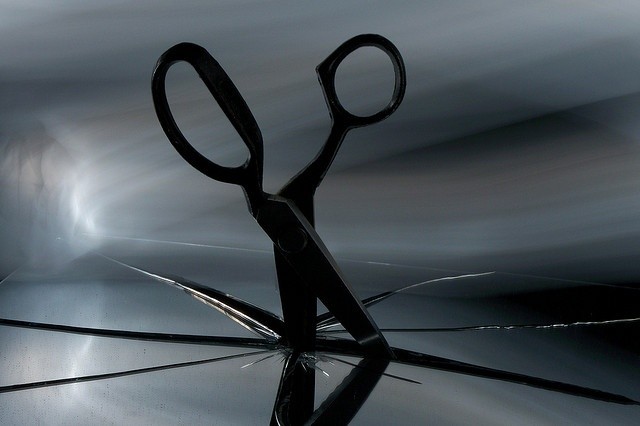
151;34;407;357
266;358;390;426
